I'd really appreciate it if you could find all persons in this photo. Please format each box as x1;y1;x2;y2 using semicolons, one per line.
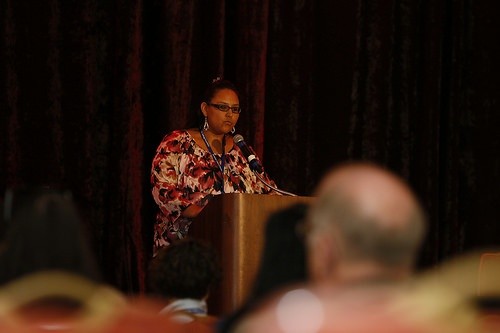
149;79;283;260
151;162;484;333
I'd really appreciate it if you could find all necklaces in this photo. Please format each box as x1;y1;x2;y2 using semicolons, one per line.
199;127;226;175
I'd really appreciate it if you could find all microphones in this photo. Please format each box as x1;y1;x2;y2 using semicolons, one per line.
233;134;263;174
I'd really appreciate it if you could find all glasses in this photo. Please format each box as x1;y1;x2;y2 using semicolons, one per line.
206;102;241;115
